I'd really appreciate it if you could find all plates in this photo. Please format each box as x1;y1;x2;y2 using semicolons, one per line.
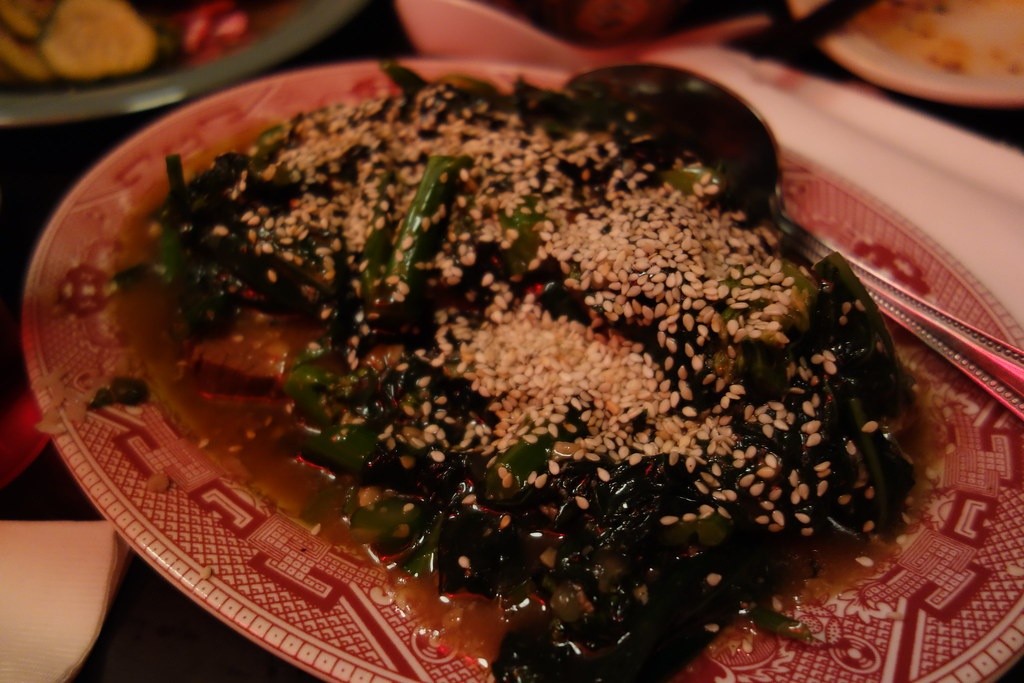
0;0;364;135
13;58;1024;683
788;0;1024;115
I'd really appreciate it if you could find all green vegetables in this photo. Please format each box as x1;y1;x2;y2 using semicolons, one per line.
115;64;913;683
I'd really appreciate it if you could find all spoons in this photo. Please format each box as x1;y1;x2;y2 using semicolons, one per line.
396;1;775;59
566;60;1024;442
